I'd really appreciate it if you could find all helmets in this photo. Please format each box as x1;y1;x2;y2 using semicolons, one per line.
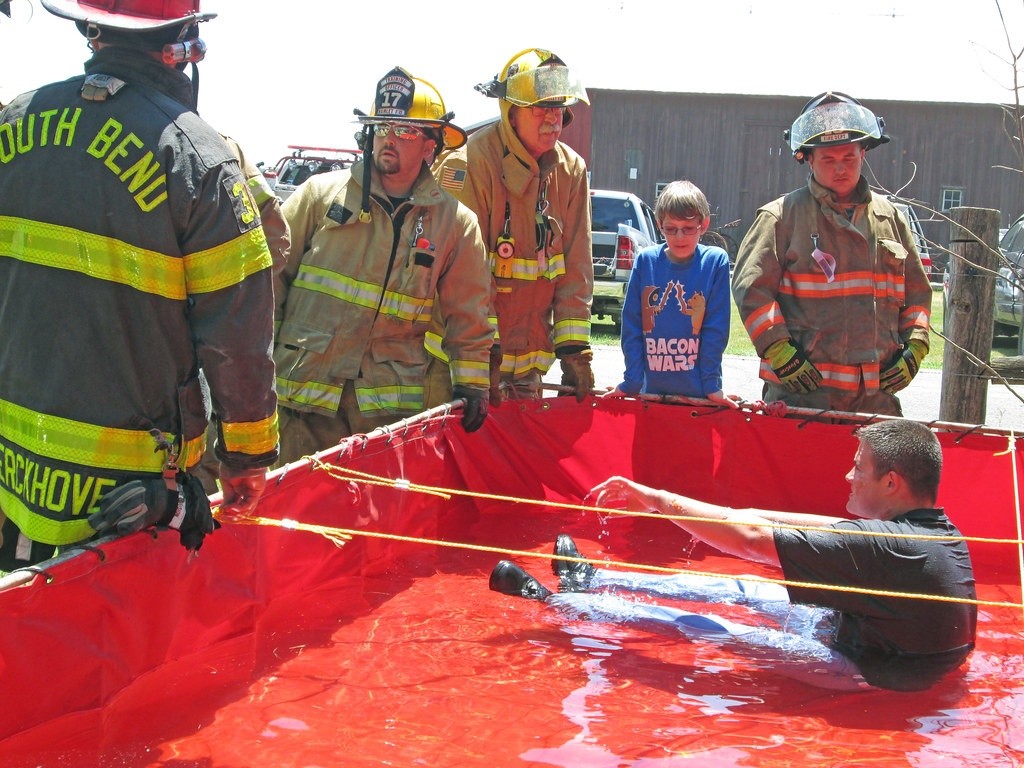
783;92;891;165
345;66;468;150
473;46;592;109
40;0;217;47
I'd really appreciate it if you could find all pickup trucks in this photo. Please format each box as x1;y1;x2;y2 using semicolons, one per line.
589;189;664;331
263;145;362;201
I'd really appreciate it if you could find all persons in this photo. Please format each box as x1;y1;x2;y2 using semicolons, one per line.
270;66;495;473
731;87;931;424
0;0;281;586
424;47;593;413
489;420;977;691
185;127;291;494
601;181;743;408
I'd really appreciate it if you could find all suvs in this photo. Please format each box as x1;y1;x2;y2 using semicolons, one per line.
897;203;932;280
994;215;1024;350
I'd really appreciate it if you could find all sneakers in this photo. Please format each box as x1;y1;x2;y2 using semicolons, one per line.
490;560;551;601
552;534;595;594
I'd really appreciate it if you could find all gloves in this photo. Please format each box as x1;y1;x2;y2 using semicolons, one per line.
86;472;223;564
765;339;823;394
556;348;595;402
877;338;928;395
487;347;504;406
461;387;490;432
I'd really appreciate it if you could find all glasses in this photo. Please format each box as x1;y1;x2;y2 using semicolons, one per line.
372;121;428;141
659;222;702;235
